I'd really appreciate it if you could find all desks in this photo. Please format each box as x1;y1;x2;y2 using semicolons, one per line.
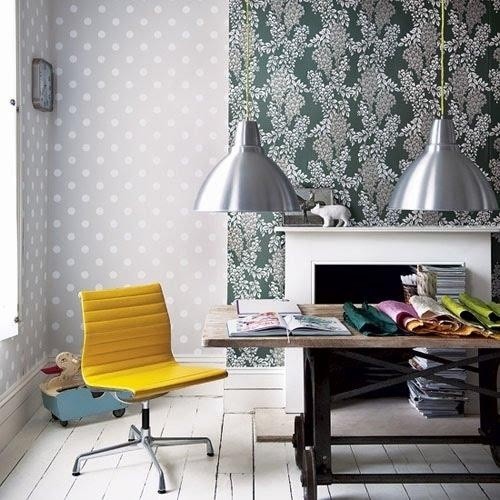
201;301;499;500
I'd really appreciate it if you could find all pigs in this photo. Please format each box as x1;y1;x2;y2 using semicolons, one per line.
310;201;353;228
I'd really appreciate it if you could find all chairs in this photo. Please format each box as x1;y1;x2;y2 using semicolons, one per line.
68;282;229;495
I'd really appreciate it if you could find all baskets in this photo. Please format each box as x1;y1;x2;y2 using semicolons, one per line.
402;281;418;303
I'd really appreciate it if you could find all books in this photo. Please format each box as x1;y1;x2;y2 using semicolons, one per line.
226;311;353;337
407;262;470;420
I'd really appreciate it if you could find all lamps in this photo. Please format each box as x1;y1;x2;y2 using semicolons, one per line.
190;1;302;216
388;0;499;214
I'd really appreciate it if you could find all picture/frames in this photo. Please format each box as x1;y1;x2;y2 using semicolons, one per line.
281;187;337;228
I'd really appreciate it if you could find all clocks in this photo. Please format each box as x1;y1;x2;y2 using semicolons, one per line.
31;56;55;113
